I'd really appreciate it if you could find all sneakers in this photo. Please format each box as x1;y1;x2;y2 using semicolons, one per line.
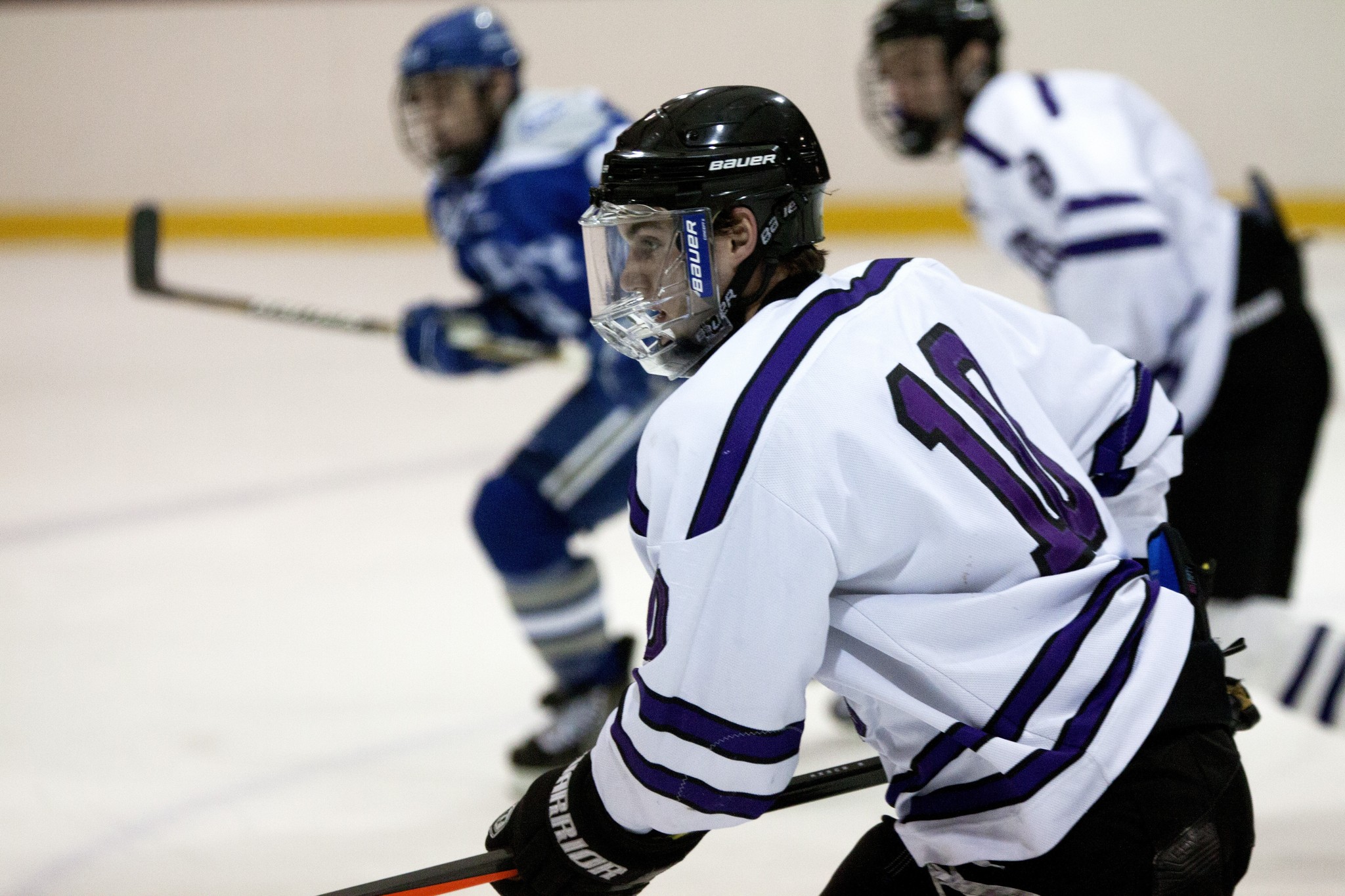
507;636;635;769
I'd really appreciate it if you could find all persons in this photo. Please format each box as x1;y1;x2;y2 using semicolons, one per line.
392;10;688;799
861;0;1345;736
483;87;1257;896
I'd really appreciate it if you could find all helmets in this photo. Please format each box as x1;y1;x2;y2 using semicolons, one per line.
391;6;527;173
856;0;1009;162
579;84;832;379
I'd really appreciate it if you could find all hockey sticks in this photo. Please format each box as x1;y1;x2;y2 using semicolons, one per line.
321;752;889;896
127;201;544;366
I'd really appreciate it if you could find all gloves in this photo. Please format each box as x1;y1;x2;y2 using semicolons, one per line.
483;757;707;896
406;298;549;378
